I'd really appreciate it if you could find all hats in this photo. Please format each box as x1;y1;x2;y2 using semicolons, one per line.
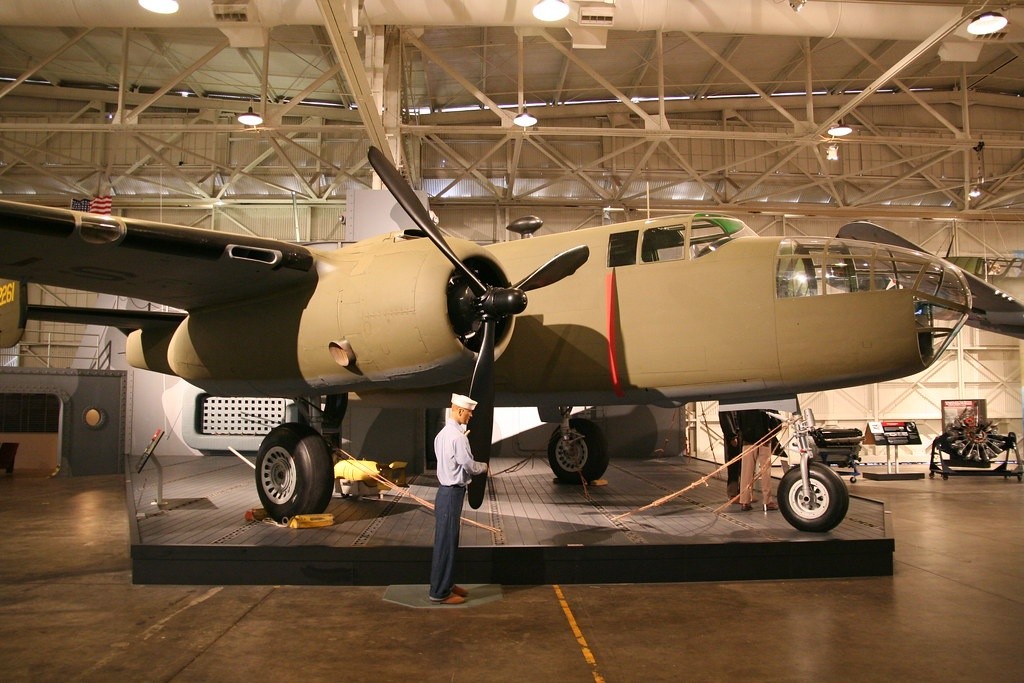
451;393;478;411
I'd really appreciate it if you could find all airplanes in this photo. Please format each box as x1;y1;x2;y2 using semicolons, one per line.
0;143;1024;534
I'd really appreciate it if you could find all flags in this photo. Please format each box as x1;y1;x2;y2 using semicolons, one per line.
72;195;112;215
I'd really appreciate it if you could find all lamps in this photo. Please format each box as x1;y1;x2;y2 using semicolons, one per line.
969;186;980;197
973;141;984;152
829;119;853;136
533;0;570;21
513;108;537;126
967;12;1009;36
236;100;265;126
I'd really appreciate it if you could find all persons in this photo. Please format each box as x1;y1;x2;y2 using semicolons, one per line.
430;393;488;604
718;411;740;504
737;410;782;511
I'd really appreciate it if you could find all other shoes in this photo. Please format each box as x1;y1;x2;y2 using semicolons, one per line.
763;502;778;511
742;502;753;511
431;594;464;604
451;586;471;596
730;495;758;504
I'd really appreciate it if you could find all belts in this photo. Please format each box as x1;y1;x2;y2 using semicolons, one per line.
443;485;463;488
